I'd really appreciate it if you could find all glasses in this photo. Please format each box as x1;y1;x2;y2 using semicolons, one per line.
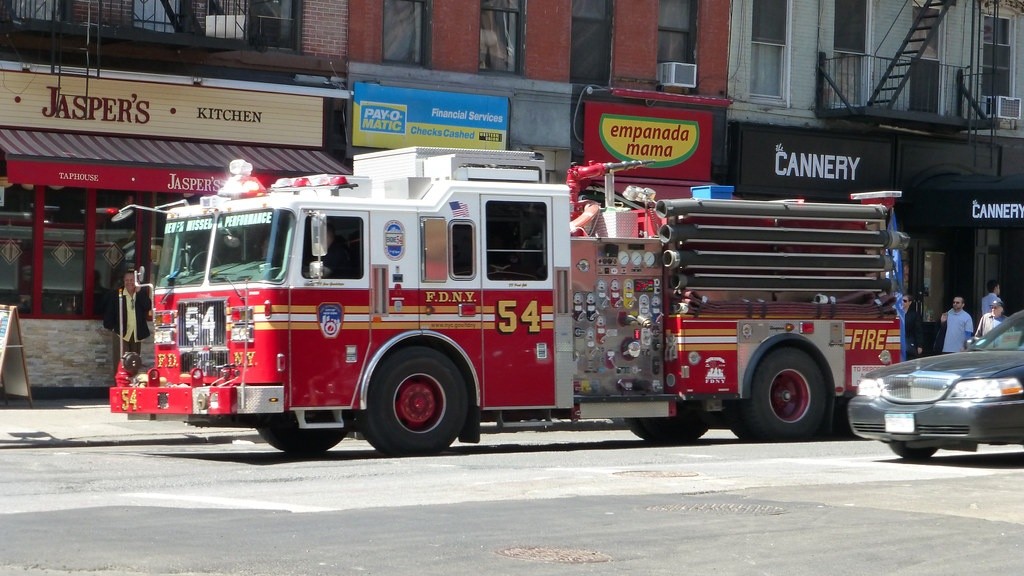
991;306;1000;309
903;300;911;303
130;298;134;310
952;301;962;304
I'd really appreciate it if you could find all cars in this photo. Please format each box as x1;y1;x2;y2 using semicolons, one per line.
846;310;1024;461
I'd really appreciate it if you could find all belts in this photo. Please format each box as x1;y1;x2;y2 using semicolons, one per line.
908;343;915;347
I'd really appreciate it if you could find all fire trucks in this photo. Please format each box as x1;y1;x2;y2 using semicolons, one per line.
109;145;907;459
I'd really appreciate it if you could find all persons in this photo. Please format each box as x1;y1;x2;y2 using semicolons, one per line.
103;269;151;355
303;224;353;279
903;295;923;361
245;237;283;267
982;280;1001;316
975;301;1014;349
941;296;973;355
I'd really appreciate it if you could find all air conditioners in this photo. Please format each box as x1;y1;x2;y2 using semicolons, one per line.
659;61;697;88
981;95;1022;120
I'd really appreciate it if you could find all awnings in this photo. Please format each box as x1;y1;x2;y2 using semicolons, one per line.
0;128;354;195
587;177;743;205
903;174;1024;231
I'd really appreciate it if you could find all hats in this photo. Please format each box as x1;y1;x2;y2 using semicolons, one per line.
989;296;1004;306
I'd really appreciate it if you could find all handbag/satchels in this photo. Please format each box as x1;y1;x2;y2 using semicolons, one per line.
933;320;947;353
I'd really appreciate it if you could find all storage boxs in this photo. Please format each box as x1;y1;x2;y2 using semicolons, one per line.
591;210;638;238
689;185;735;200
